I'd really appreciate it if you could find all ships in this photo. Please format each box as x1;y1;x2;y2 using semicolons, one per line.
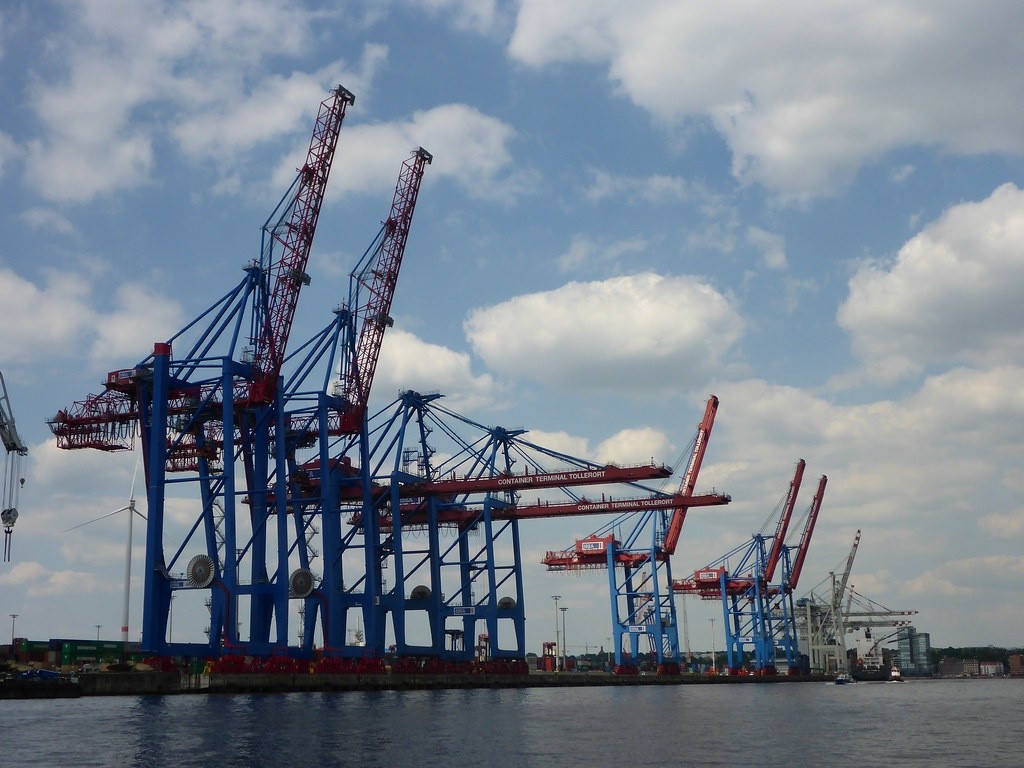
852;655;892;681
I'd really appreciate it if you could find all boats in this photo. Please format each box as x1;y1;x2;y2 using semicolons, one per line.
836;673;852;684
